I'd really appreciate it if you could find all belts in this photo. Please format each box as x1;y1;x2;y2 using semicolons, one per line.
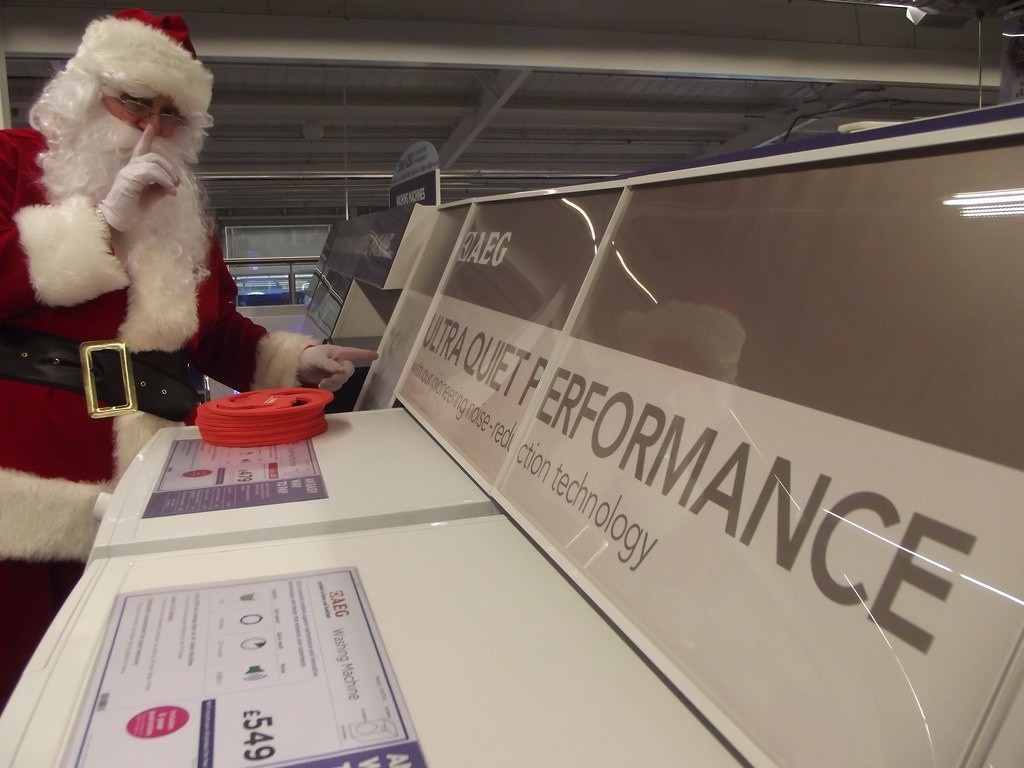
0;324;204;421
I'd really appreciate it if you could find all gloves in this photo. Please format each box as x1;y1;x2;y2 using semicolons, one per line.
96;124;182;232
296;344;379;393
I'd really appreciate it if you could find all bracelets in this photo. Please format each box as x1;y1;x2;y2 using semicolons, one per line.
96;207;107;228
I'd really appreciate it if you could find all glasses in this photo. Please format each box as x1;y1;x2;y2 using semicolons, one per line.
115;96;185;127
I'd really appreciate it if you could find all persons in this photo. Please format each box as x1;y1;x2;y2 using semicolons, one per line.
0;7;379;768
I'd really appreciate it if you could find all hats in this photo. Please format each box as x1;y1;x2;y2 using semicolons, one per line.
74;8;214;120
